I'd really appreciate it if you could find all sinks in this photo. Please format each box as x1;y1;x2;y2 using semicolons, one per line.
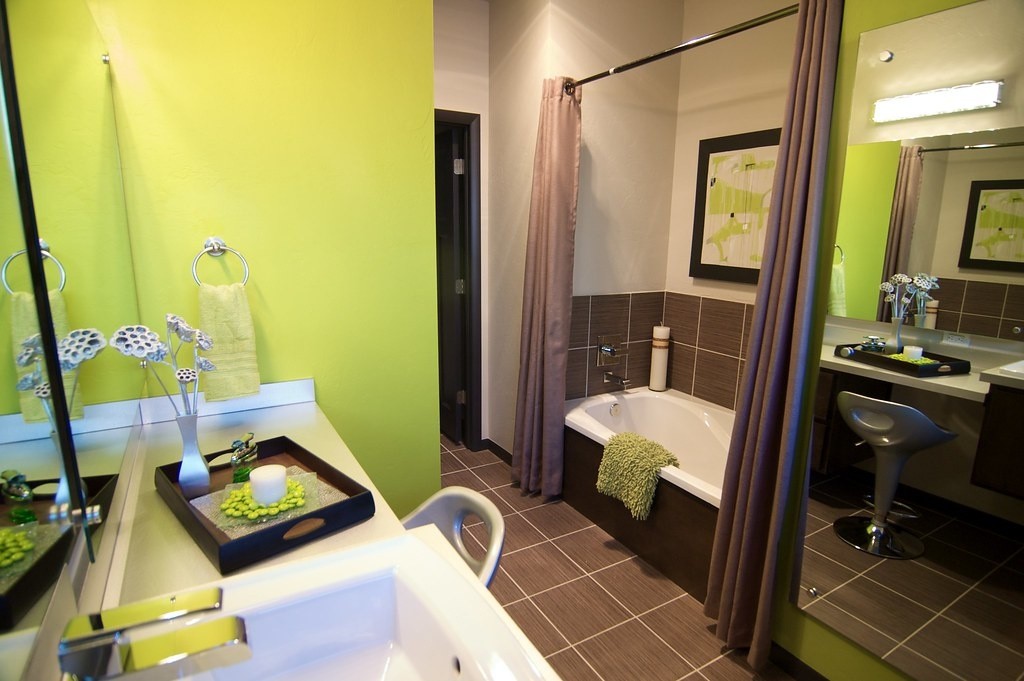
155;524;559;681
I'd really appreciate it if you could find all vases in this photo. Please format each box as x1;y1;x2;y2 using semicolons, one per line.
914;314;926;327
885;316;905;355
49;428;89;512
176;414;212;499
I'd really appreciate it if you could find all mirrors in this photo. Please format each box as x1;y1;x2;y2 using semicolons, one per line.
1;4;145;681
788;126;1024;681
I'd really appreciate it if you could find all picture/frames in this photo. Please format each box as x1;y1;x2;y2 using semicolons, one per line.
958;179;1024;272
689;125;781;286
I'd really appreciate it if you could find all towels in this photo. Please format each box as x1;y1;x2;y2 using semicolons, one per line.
196;282;262;404
596;432;680;519
8;288;86;424
826;264;847;318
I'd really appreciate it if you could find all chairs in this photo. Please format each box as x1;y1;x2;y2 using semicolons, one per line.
832;390;960;564
396;486;505;589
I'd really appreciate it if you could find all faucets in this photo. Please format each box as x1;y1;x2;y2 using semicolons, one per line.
56;585;255;681
603;371;632;388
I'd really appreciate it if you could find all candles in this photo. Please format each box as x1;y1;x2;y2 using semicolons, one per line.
649;321;670;391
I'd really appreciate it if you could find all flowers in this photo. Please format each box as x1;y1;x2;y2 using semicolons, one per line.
880;272;932;318
909;273;940;315
16;327;108;431
111;313;216;416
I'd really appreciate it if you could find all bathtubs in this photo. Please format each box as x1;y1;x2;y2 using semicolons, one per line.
562;383;735;603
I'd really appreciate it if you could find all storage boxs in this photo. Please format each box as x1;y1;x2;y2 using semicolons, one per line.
833;344;971;378
154;435;375;576
1;475;117;632
